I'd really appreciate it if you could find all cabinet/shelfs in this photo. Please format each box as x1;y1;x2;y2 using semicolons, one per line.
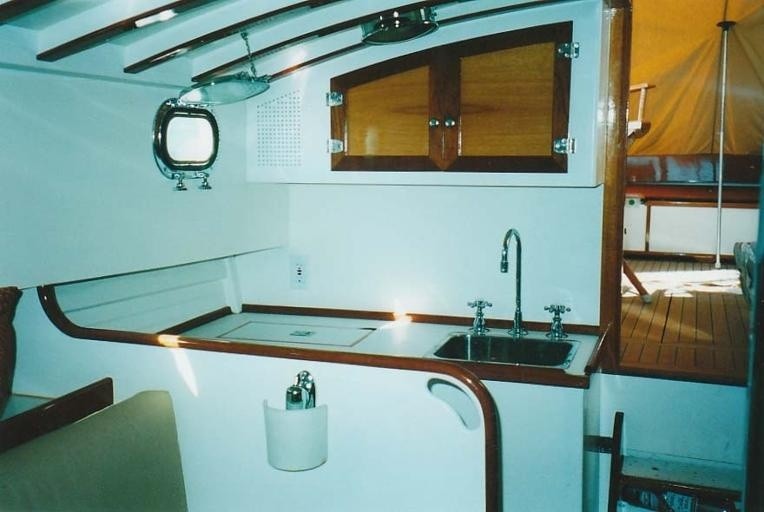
325;21;575;175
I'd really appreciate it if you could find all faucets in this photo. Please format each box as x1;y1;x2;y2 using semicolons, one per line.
499;227;528;336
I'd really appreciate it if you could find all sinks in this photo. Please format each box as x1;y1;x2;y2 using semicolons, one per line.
426;330;579;370
